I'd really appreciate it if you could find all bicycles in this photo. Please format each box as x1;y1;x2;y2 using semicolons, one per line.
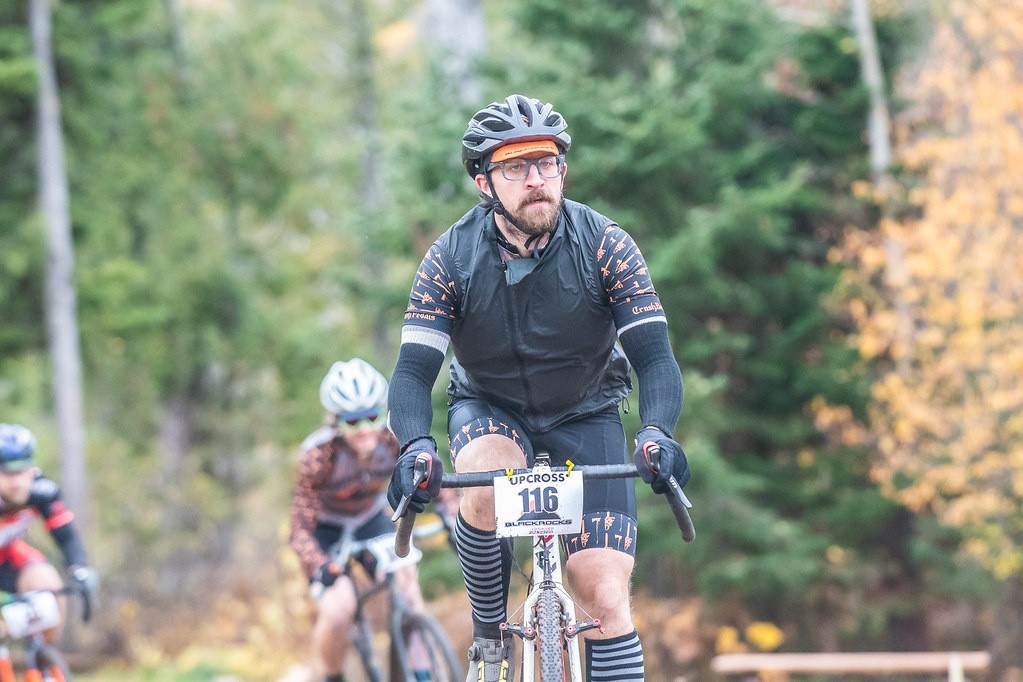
309;516;464;682
0;584;84;682
391;439;698;682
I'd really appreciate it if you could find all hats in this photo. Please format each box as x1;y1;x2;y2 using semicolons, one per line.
487;141;561;170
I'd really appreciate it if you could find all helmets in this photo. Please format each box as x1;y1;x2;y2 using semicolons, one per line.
320;358;389;421
0;424;33;463
461;94;571;180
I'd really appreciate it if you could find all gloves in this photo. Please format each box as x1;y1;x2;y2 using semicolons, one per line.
633;429;690;494
318;562;338;587
387;438;443;518
72;567;98;598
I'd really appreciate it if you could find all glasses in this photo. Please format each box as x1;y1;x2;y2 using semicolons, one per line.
487;154;564;181
0;458;35;472
338;406;388;429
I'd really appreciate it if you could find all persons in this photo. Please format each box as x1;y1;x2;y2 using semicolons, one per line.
289;358;435;682
0;425;98;682
387;93;691;682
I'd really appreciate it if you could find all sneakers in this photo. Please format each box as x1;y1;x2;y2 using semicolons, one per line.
465;635;516;682
